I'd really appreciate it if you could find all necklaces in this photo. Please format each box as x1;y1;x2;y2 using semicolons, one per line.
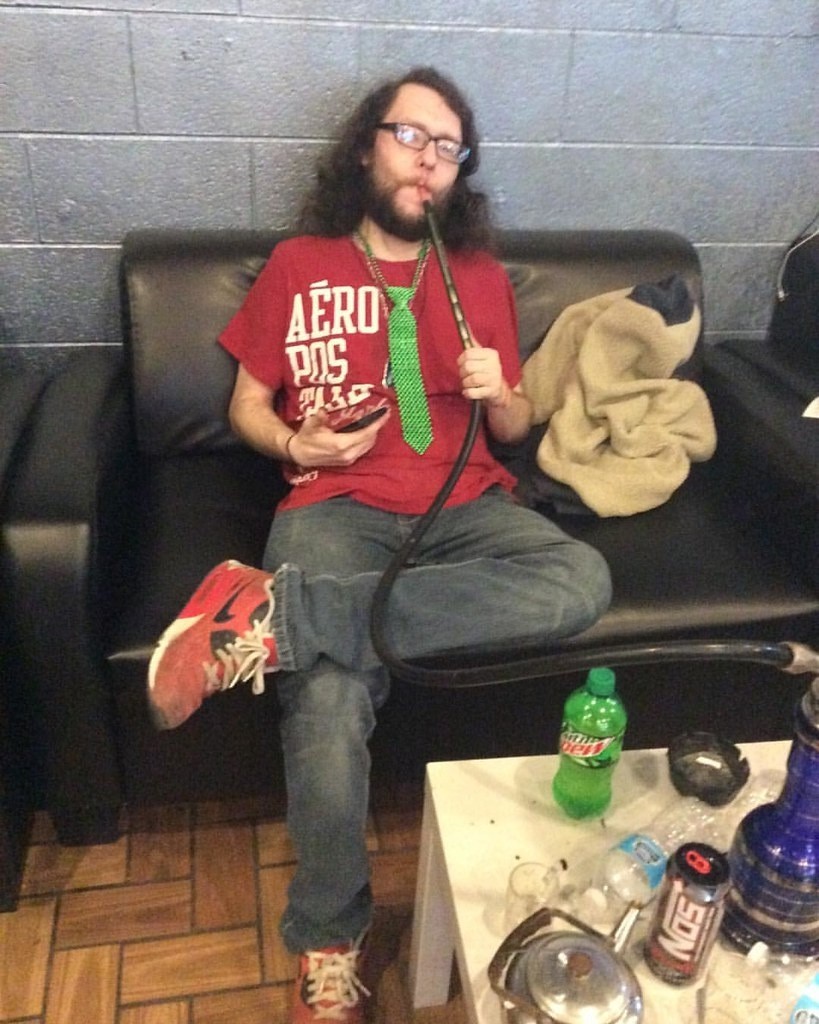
364;218;432;387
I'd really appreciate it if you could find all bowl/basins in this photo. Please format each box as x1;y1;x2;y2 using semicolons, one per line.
667;731;751;804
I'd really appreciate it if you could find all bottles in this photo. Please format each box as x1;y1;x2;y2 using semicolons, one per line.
550;665;628;822
584;770;787;916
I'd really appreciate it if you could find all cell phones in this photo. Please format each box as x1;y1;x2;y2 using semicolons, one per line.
335;407;388;433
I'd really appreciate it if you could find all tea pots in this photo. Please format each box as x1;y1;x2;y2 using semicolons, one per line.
489;901;646;1024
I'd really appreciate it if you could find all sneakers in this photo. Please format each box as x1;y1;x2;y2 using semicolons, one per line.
146;560;280;729
293;931;373;1024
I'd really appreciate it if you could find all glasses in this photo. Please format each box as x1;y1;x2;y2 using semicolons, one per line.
374;122;470;164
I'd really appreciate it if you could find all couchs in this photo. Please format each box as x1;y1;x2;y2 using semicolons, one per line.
0;225;819;911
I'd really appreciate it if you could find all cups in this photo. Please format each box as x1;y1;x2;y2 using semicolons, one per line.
504;862;560;935
692;948;819;1024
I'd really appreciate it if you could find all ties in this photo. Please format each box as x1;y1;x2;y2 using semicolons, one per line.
383;285;434;455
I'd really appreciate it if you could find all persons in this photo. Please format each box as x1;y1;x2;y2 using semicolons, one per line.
147;67;614;1024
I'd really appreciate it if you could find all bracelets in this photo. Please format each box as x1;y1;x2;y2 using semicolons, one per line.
286;435;294;461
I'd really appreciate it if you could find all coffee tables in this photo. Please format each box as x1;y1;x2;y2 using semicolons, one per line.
407;741;819;1024
